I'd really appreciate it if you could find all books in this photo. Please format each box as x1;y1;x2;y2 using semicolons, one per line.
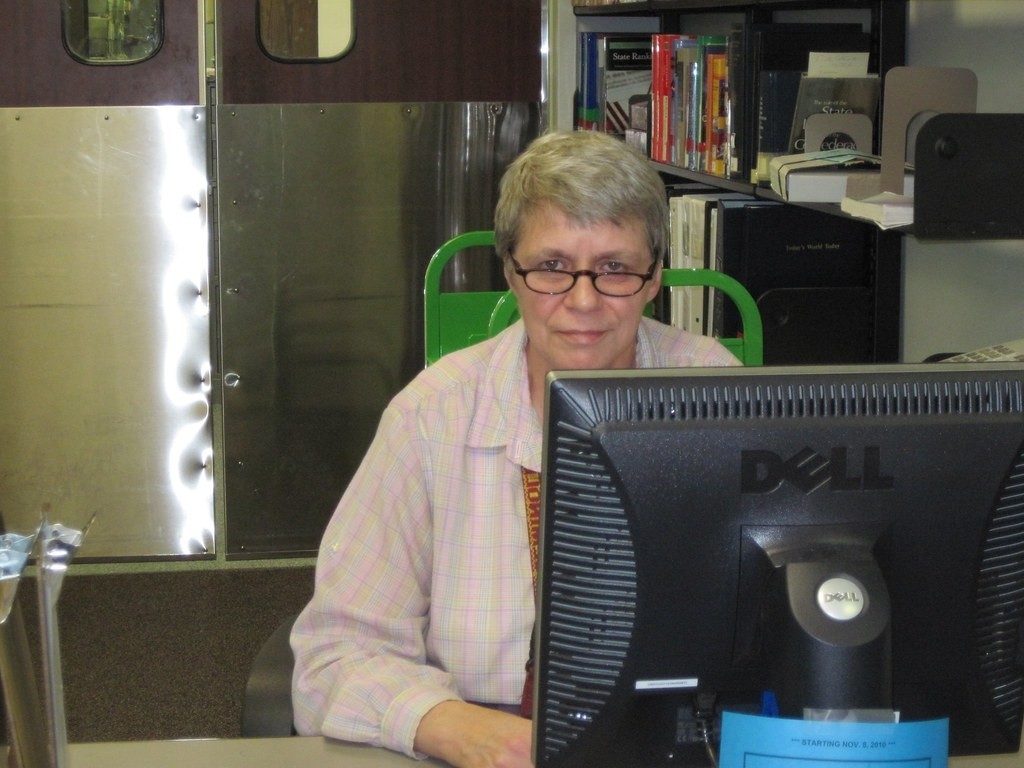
574;15;883;340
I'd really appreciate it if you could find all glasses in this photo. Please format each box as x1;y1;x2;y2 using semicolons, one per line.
508;245;660;298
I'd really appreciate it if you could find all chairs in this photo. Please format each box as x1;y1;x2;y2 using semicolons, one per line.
424;232;523;367
490;267;764;368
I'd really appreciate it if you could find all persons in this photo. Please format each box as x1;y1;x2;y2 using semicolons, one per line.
287;128;765;767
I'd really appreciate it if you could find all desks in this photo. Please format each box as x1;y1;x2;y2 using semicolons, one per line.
64;735;462;767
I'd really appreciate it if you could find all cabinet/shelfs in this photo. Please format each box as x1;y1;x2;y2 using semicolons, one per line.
569;1;911;366
